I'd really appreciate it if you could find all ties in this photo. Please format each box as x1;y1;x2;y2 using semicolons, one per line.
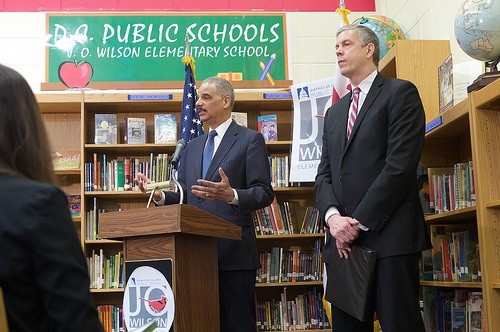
346;87;361;141
201;130;217;180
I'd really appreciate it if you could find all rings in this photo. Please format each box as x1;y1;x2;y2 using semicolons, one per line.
206;192;210;197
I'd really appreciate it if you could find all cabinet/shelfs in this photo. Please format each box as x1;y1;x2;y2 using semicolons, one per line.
34;39;500;332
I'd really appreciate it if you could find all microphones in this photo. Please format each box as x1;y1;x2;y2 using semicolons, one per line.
172;139;186;165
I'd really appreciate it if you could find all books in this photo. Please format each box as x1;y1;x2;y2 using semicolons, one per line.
65;113;328;332
417;160;483;332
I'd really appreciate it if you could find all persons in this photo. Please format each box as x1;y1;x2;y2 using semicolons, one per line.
133;77;274;332
313;24;434;332
0;63;103;332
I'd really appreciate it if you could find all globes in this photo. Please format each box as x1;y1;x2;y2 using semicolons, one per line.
351;14;406;62
454;0;500;93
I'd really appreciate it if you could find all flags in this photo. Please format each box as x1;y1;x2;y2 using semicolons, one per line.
170;45;204;166
